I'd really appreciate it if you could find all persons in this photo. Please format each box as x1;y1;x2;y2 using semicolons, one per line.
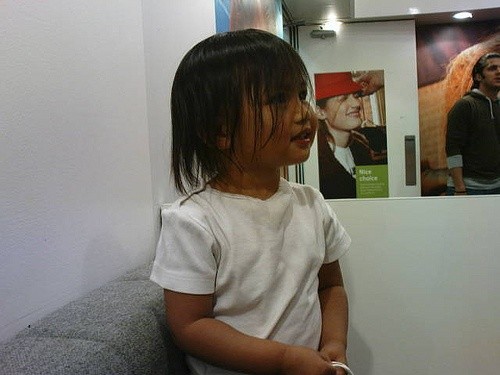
314;71;376;199
150;27;353;375
446;51;500;195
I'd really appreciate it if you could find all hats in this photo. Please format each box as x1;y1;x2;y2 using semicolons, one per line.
315;72;362;101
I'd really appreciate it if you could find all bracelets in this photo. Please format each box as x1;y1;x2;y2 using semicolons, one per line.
455;190;467;193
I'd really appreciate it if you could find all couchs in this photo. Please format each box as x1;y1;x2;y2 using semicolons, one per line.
0;254;197;375
